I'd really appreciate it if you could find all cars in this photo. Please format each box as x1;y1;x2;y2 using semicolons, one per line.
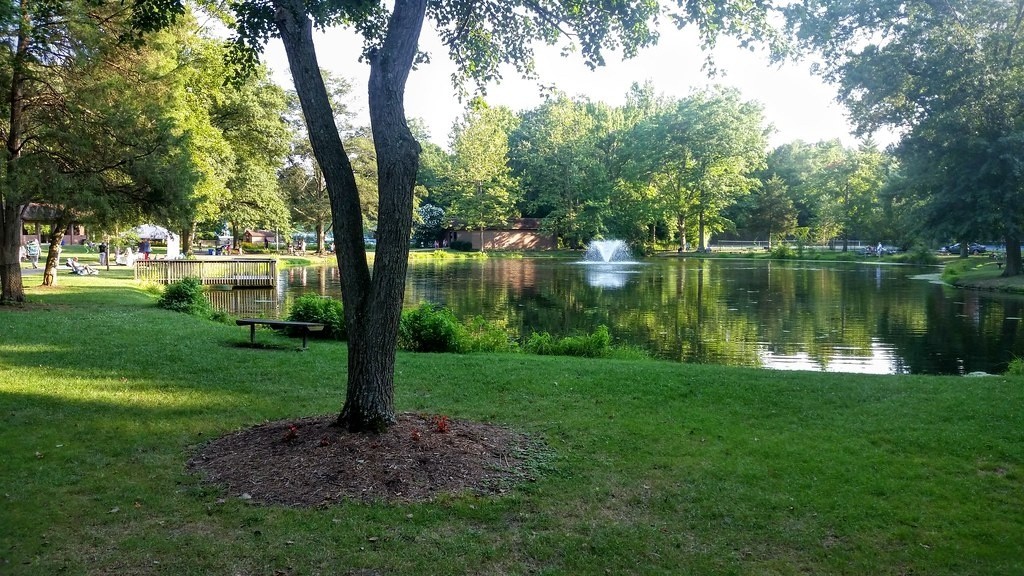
944;239;986;254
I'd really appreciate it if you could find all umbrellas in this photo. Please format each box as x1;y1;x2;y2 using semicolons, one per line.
120;224;180;239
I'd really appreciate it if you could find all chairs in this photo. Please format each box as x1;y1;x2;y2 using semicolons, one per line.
67;258;98;276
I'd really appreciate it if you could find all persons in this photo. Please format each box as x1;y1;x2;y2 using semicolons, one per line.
265;237;268;250
226;239;231;255
30;239;41;269
140;239;152;260
99;242;106;266
325;243;336;253
72;257;98;274
866;242;884;251
301;239;305;256
433;239;447;248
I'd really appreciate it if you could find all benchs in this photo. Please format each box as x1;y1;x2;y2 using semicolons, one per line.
864;250;884;258
237;318;325;348
996;262;1003;269
